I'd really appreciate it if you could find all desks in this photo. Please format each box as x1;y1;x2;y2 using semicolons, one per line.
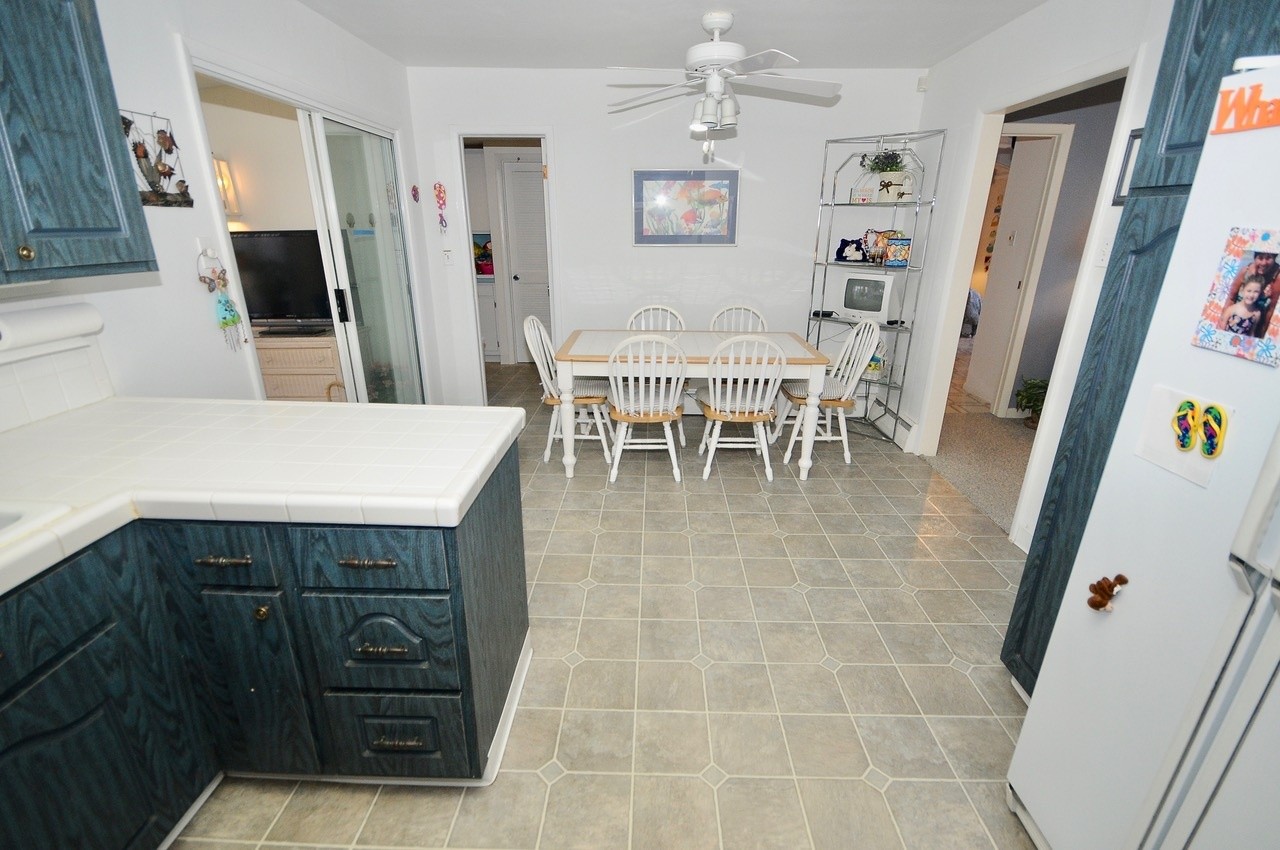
555;329;830;481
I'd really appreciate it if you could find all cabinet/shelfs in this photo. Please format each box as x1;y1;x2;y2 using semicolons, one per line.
1;524;475;850
807;129;947;443
1;1;161;275
251;327;346;402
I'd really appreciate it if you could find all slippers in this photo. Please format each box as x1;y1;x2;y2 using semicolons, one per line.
1198;403;1226;459
1170;399;1199;452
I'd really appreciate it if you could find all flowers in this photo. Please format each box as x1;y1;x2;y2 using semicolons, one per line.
371;358;416;403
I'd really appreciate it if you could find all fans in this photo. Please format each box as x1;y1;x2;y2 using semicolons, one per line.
605;13;842;106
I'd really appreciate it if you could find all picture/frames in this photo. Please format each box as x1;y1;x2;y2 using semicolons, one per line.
633;169;740;246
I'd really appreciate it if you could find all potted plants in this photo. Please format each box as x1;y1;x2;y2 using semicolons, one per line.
880;228;911;267
862;153;915;201
1013;374;1050;429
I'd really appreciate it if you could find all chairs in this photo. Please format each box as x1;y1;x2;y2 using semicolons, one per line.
626;304;689;388
609;335;687;484
698;336;786;480
523;315;616;464
710;307;771;439
771;319;881;465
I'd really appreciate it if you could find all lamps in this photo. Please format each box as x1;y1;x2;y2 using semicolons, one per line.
688;95;739;155
212;152;241;222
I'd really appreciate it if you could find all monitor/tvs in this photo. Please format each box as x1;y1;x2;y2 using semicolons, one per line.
839;272;902;327
230;229;365;338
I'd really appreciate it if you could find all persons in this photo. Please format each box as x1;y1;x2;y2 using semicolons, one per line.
1216;251;1280;339
